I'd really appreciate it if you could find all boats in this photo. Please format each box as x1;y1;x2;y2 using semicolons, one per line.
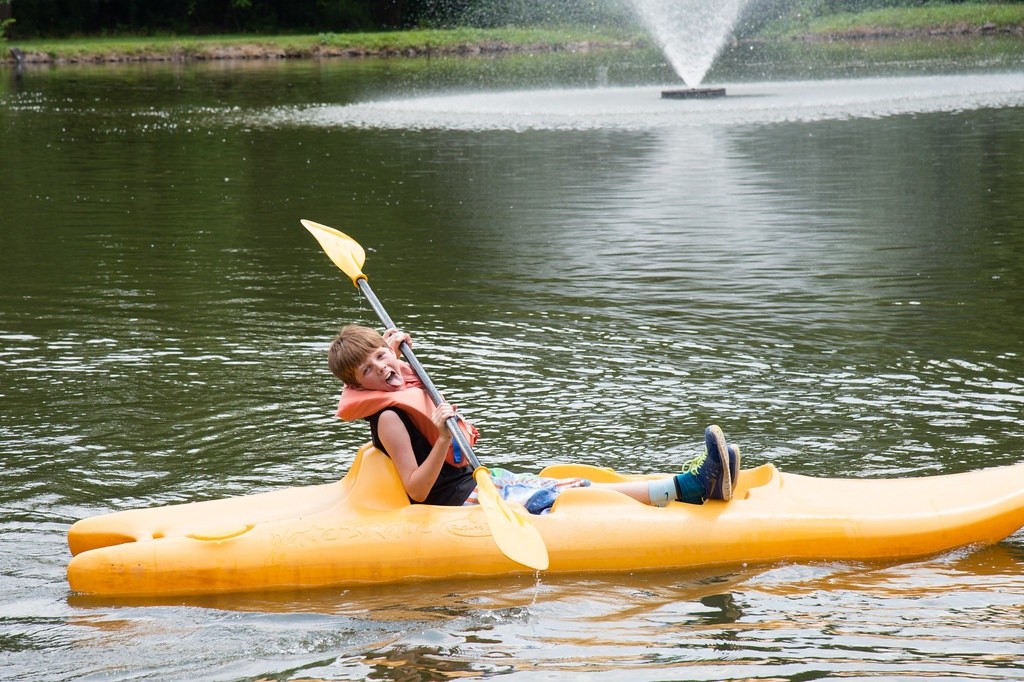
62;437;1024;603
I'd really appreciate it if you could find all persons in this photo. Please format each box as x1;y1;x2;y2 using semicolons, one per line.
328;324;741;516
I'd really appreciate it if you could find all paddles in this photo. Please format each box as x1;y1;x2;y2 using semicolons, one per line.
300;219;549;572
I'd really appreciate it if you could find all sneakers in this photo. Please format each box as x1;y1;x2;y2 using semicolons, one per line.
682;424;740;501
